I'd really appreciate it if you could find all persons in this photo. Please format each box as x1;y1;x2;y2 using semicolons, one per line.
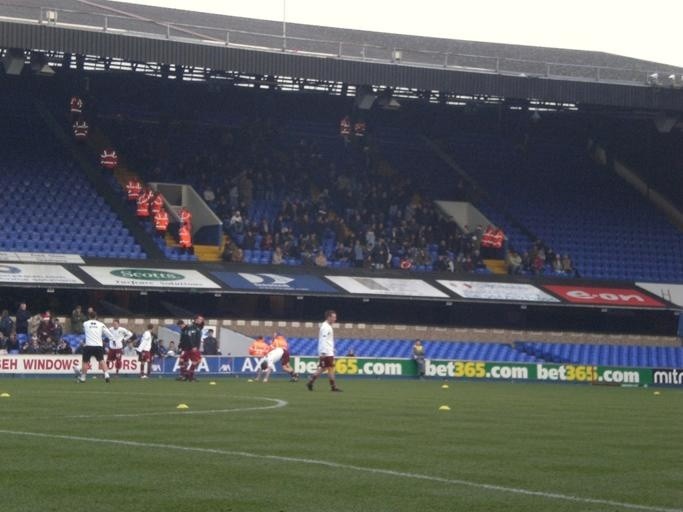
172;318;193;381
410;338;426;382
136;324;156;379
345;349;354;356
247;335;269;356
75;310;115;385
269;329;288;352
0;300;220;360
254;347;299;383
69;92;575;282
181;313;203;384
305;308;343;392
106;318;133;379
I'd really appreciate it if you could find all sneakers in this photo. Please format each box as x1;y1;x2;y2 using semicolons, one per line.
106;378;110;383
75;378;85;384
141;374;148;378
177;375;199;382
290;373;298;382
331;388;342;391
307;382;313;391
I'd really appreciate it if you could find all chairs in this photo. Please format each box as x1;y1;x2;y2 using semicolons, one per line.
0;105;683;367
